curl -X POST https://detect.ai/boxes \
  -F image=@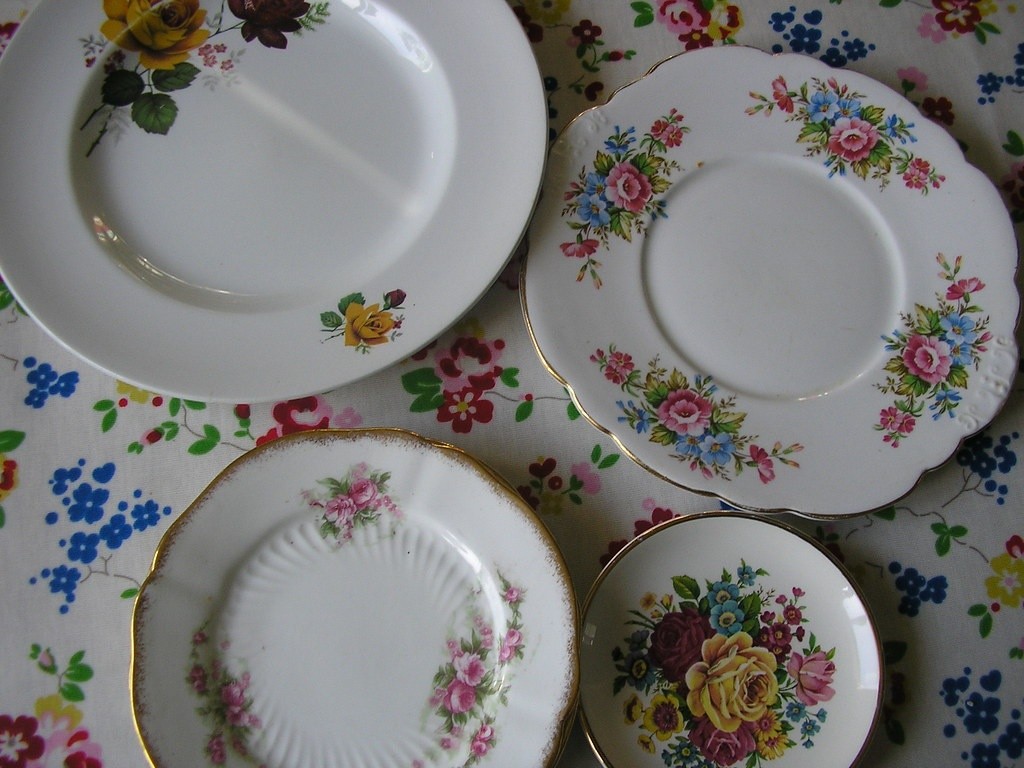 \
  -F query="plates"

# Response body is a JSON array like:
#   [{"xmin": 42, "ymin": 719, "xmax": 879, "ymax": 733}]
[
  {"xmin": 131, "ymin": 428, "xmax": 581, "ymax": 768},
  {"xmin": 574, "ymin": 510, "xmax": 884, "ymax": 768},
  {"xmin": 0, "ymin": 0, "xmax": 551, "ymax": 404},
  {"xmin": 517, "ymin": 44, "xmax": 1021, "ymax": 521}
]
